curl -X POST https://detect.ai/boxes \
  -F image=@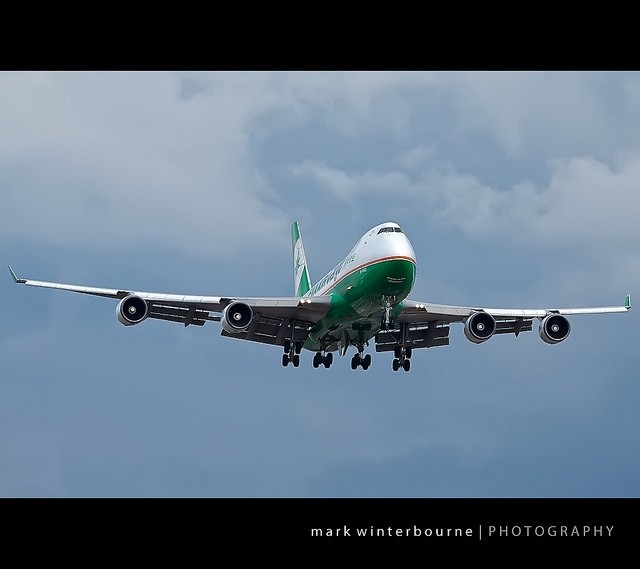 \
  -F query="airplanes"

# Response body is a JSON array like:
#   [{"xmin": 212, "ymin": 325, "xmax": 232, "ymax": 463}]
[{"xmin": 7, "ymin": 222, "xmax": 631, "ymax": 373}]
